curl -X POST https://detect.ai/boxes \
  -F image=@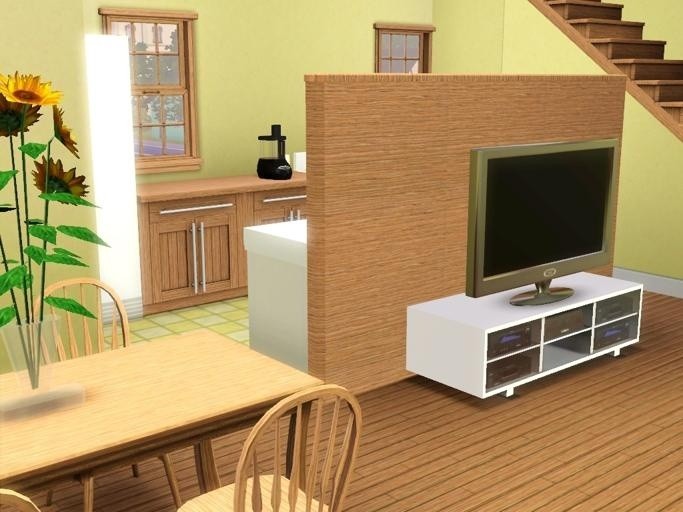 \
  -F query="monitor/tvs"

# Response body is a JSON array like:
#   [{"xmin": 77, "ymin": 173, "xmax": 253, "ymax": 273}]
[{"xmin": 466, "ymin": 137, "xmax": 618, "ymax": 306}]
